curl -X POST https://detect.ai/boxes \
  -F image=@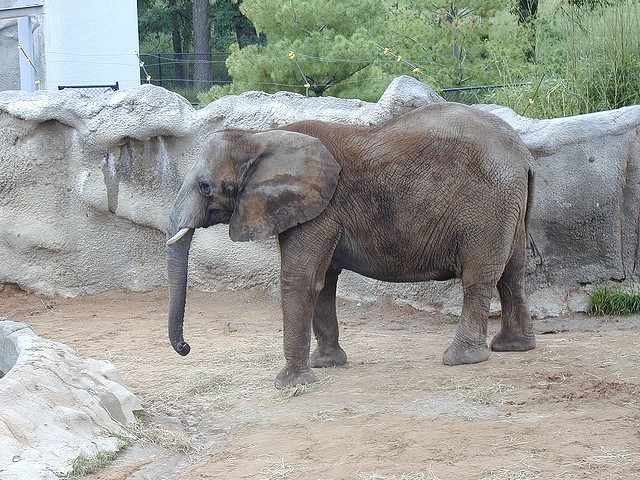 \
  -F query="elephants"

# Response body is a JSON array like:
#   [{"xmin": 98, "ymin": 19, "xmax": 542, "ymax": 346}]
[{"xmin": 165, "ymin": 102, "xmax": 536, "ymax": 391}]
